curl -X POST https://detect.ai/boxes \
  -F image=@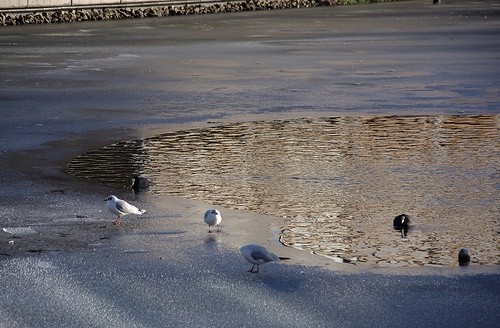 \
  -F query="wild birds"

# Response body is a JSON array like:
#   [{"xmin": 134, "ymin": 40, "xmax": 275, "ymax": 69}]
[
  {"xmin": 104, "ymin": 196, "xmax": 145, "ymax": 224},
  {"xmin": 240, "ymin": 245, "xmax": 292, "ymax": 273},
  {"xmin": 130, "ymin": 173, "xmax": 152, "ymax": 189},
  {"xmin": 393, "ymin": 212, "xmax": 417, "ymax": 229},
  {"xmin": 204, "ymin": 210, "xmax": 222, "ymax": 233},
  {"xmin": 457, "ymin": 248, "xmax": 476, "ymax": 265}
]
